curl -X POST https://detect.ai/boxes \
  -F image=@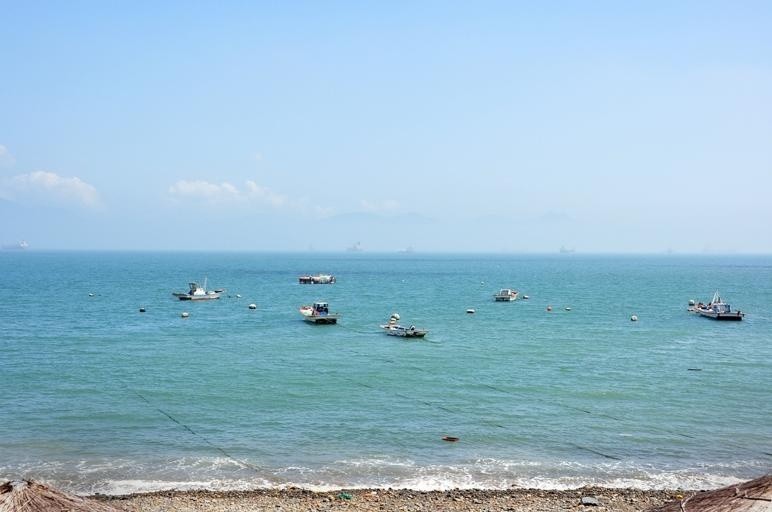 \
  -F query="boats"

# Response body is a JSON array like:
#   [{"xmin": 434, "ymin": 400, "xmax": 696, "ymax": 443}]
[
  {"xmin": 379, "ymin": 313, "xmax": 429, "ymax": 337},
  {"xmin": 298, "ymin": 271, "xmax": 337, "ymax": 285},
  {"xmin": 298, "ymin": 301, "xmax": 338, "ymax": 324},
  {"xmin": 688, "ymin": 301, "xmax": 744, "ymax": 320},
  {"xmin": 172, "ymin": 274, "xmax": 227, "ymax": 301},
  {"xmin": 493, "ymin": 289, "xmax": 520, "ymax": 301}
]
